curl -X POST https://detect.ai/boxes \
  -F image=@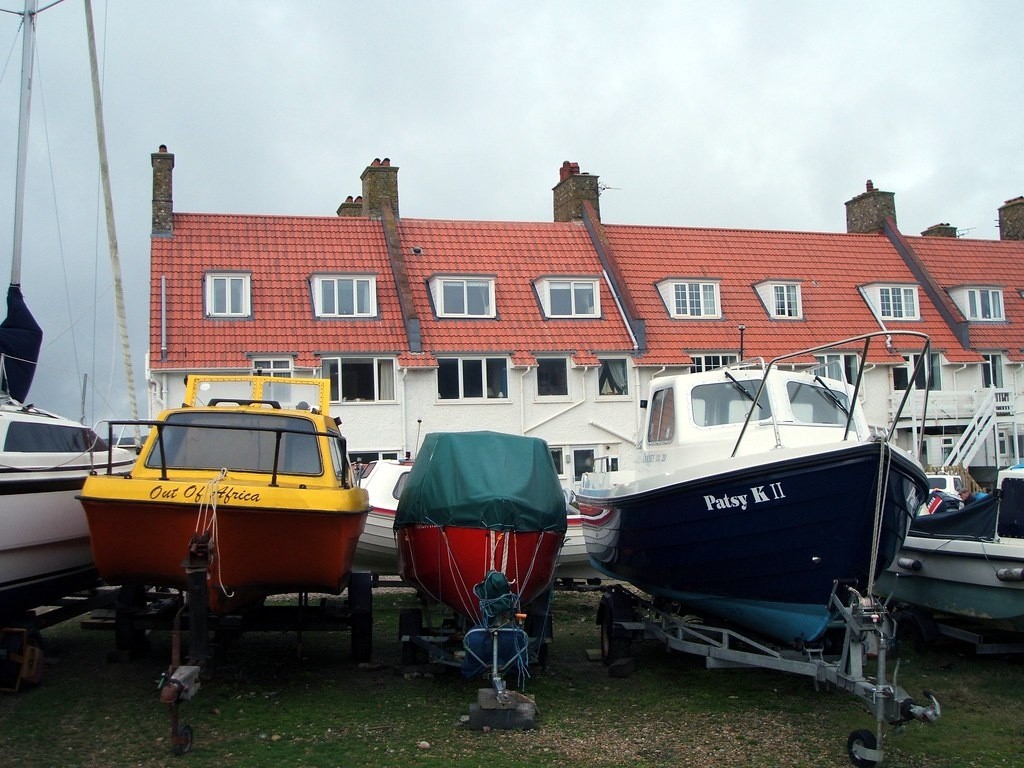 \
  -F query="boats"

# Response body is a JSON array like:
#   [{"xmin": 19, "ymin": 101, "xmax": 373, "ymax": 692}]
[
  {"xmin": 864, "ymin": 462, "xmax": 1024, "ymax": 633},
  {"xmin": 393, "ymin": 430, "xmax": 568, "ymax": 634},
  {"xmin": 352, "ymin": 451, "xmax": 618, "ymax": 579},
  {"xmin": 568, "ymin": 328, "xmax": 933, "ymax": 650},
  {"xmin": 0, "ymin": 393, "xmax": 144, "ymax": 617},
  {"xmin": 72, "ymin": 369, "xmax": 376, "ymax": 615}
]
